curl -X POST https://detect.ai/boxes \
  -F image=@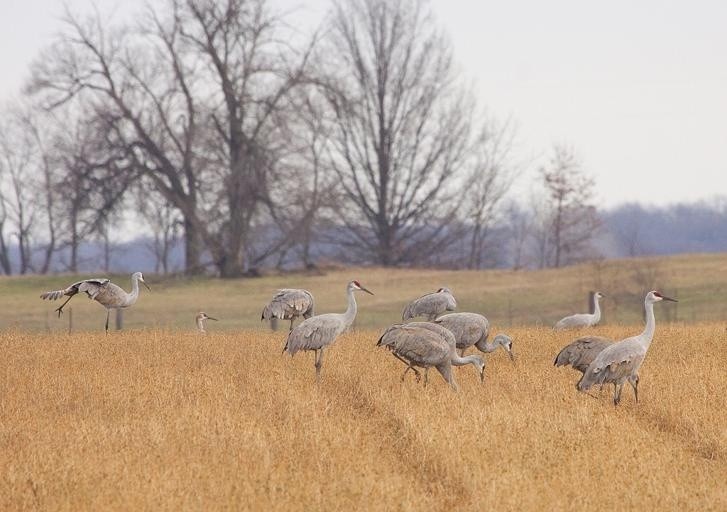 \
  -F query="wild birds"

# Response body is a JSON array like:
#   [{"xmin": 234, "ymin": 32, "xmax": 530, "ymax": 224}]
[
  {"xmin": 40, "ymin": 271, "xmax": 152, "ymax": 332},
  {"xmin": 194, "ymin": 312, "xmax": 220, "ymax": 334},
  {"xmin": 261, "ymin": 289, "xmax": 315, "ymax": 335},
  {"xmin": 376, "ymin": 285, "xmax": 514, "ymax": 388},
  {"xmin": 283, "ymin": 279, "xmax": 376, "ymax": 374},
  {"xmin": 553, "ymin": 289, "xmax": 680, "ymax": 408}
]
